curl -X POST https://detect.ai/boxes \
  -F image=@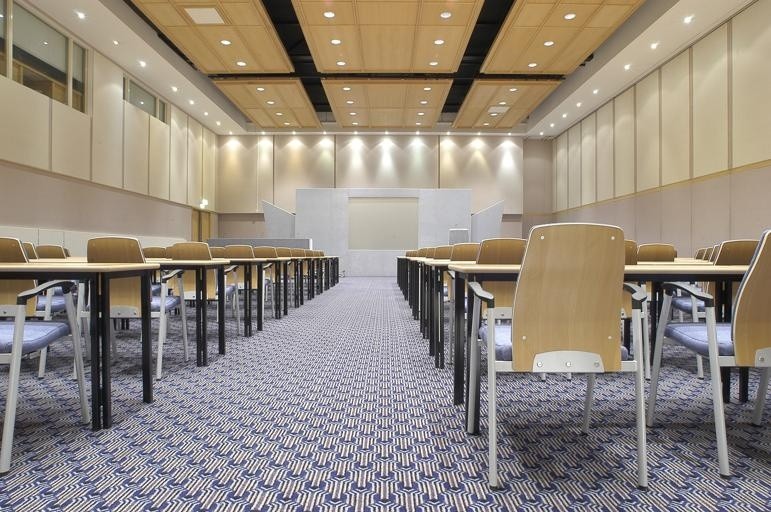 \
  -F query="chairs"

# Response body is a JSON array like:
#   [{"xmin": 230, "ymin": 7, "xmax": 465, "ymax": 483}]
[
  {"xmin": 396, "ymin": 222, "xmax": 771, "ymax": 490},
  {"xmin": 87, "ymin": 238, "xmax": 257, "ymax": 381},
  {"xmin": 252, "ymin": 246, "xmax": 339, "ymax": 332},
  {"xmin": 0, "ymin": 237, "xmax": 154, "ymax": 474}
]
